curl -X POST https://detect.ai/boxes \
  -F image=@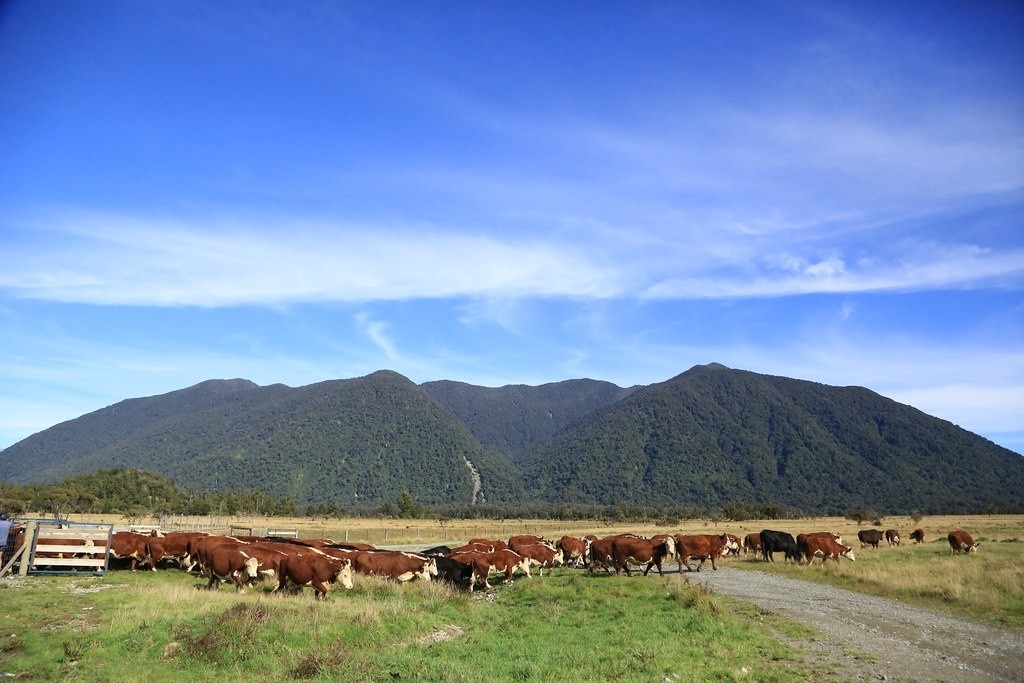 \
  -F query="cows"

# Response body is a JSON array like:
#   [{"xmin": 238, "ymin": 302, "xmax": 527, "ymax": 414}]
[{"xmin": 12, "ymin": 519, "xmax": 981, "ymax": 603}]
